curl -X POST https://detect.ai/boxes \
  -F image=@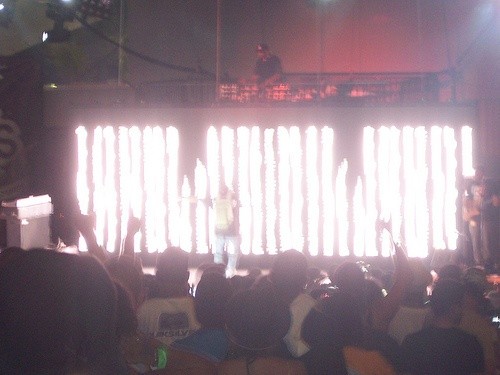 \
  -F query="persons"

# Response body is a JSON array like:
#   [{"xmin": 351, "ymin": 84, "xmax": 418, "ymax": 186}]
[
  {"xmin": 239, "ymin": 43, "xmax": 291, "ymax": 90},
  {"xmin": 0, "ymin": 185, "xmax": 499, "ymax": 375},
  {"xmin": 459, "ymin": 169, "xmax": 498, "ymax": 268}
]
